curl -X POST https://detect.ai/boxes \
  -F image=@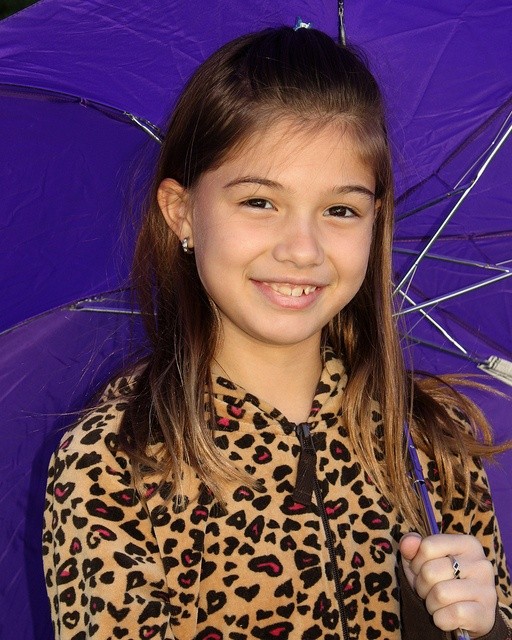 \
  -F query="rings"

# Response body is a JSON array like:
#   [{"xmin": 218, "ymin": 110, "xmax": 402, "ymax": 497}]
[{"xmin": 446, "ymin": 554, "xmax": 466, "ymax": 584}]
